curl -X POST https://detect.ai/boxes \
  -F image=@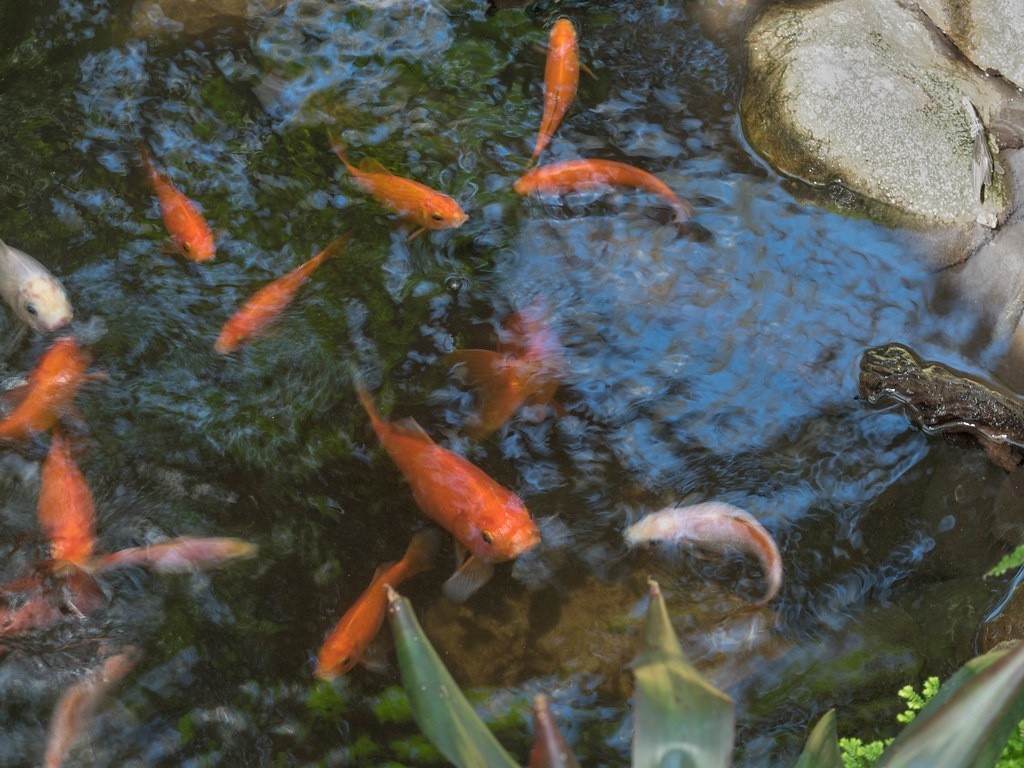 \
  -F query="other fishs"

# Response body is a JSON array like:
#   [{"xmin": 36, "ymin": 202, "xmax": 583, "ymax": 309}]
[
  {"xmin": 1, "ymin": 580, "xmax": 105, "ymax": 637},
  {"xmin": 359, "ymin": 389, "xmax": 543, "ymax": 602},
  {"xmin": 513, "ymin": 158, "xmax": 692, "ymax": 225},
  {"xmin": 0, "ymin": 337, "xmax": 87, "ymax": 441},
  {"xmin": 44, "ymin": 645, "xmax": 137, "ymax": 767},
  {"xmin": 212, "ymin": 226, "xmax": 352, "ymax": 355},
  {"xmin": 146, "ymin": 155, "xmax": 229, "ymax": 264},
  {"xmin": 622, "ymin": 500, "xmax": 784, "ymax": 605},
  {"xmin": 326, "ymin": 125, "xmax": 470, "ymax": 244},
  {"xmin": 94, "ymin": 535, "xmax": 259, "ymax": 576},
  {"xmin": 0, "ymin": 241, "xmax": 73, "ymax": 333},
  {"xmin": 442, "ymin": 298, "xmax": 565, "ymax": 442},
  {"xmin": 309, "ymin": 528, "xmax": 443, "ymax": 683},
  {"xmin": 36, "ymin": 419, "xmax": 95, "ymax": 578},
  {"xmin": 527, "ymin": 16, "xmax": 598, "ymax": 157}
]
